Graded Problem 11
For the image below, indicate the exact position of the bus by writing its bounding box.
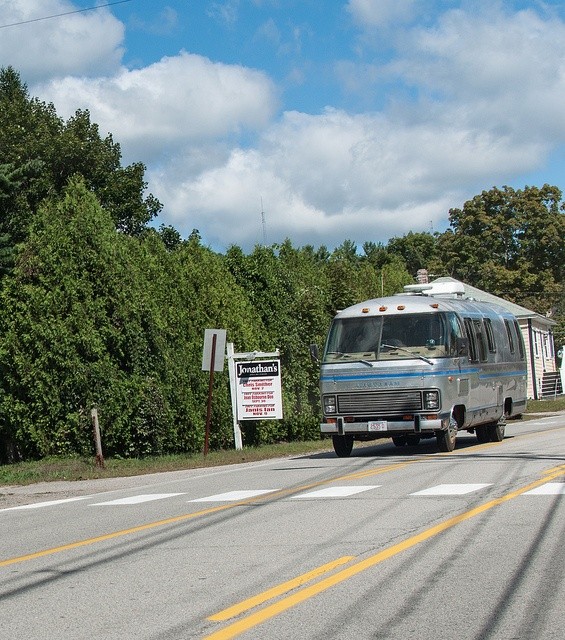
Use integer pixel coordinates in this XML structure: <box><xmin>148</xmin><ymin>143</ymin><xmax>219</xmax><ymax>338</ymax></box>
<box><xmin>319</xmin><ymin>282</ymin><xmax>527</xmax><ymax>457</ymax></box>
